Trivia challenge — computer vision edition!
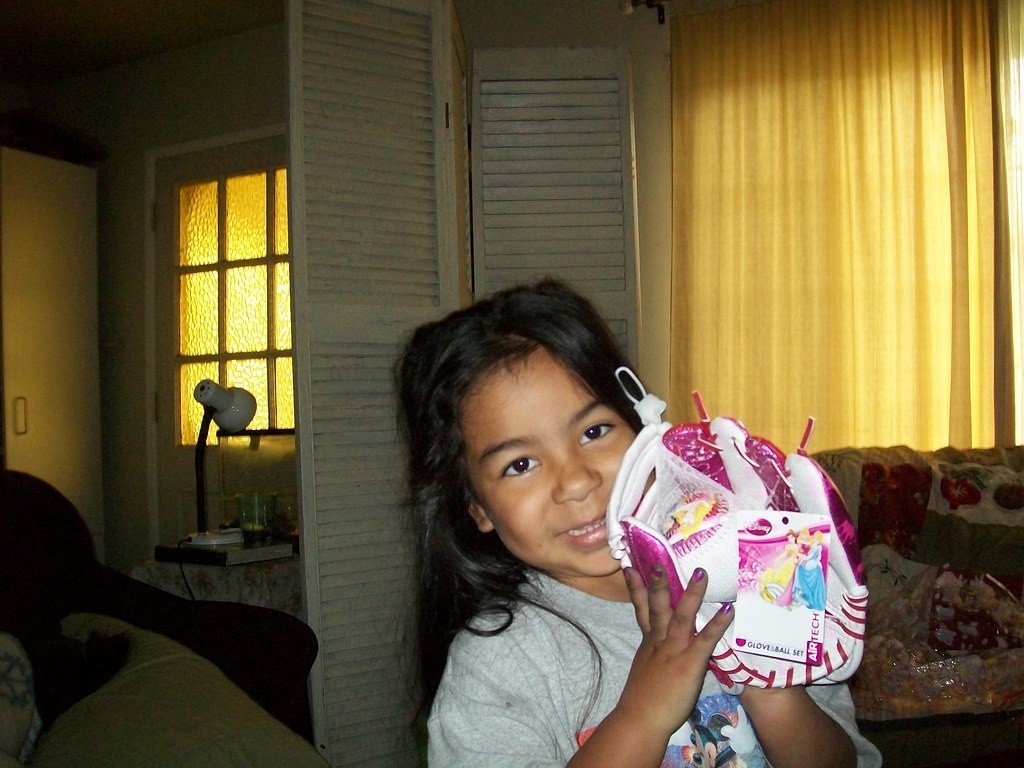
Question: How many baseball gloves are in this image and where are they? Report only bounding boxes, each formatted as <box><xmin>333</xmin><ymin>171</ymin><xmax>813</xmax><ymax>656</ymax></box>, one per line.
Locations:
<box><xmin>604</xmin><ymin>415</ymin><xmax>872</xmax><ymax>696</ymax></box>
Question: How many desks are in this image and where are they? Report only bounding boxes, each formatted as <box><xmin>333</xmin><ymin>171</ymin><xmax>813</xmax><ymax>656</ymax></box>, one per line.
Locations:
<box><xmin>143</xmin><ymin>556</ymin><xmax>302</xmax><ymax>617</ymax></box>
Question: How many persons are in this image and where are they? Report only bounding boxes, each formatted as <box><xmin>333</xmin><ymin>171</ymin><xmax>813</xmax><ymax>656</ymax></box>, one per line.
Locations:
<box><xmin>392</xmin><ymin>275</ymin><xmax>883</xmax><ymax>768</ymax></box>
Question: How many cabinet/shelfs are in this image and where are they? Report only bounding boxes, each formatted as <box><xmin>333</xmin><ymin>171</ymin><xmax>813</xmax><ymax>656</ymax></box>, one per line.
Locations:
<box><xmin>0</xmin><ymin>142</ymin><xmax>106</xmax><ymax>565</ymax></box>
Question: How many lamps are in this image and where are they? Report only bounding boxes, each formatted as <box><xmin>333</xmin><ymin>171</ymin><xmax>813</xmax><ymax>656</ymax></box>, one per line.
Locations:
<box><xmin>185</xmin><ymin>379</ymin><xmax>257</xmax><ymax>545</ymax></box>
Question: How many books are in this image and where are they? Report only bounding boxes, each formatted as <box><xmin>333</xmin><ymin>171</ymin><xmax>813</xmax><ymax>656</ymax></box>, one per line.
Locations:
<box><xmin>154</xmin><ymin>541</ymin><xmax>292</xmax><ymax>565</ymax></box>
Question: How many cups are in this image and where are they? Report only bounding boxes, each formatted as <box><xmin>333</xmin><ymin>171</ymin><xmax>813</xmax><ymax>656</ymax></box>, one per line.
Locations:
<box><xmin>274</xmin><ymin>488</ymin><xmax>297</xmax><ymax>533</ymax></box>
<box><xmin>234</xmin><ymin>492</ymin><xmax>272</xmax><ymax>546</ymax></box>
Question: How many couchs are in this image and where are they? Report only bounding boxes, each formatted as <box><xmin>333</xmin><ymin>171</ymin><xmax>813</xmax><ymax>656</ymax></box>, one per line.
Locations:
<box><xmin>808</xmin><ymin>444</ymin><xmax>1024</xmax><ymax>768</ymax></box>
<box><xmin>0</xmin><ymin>468</ymin><xmax>318</xmax><ymax>747</ymax></box>
